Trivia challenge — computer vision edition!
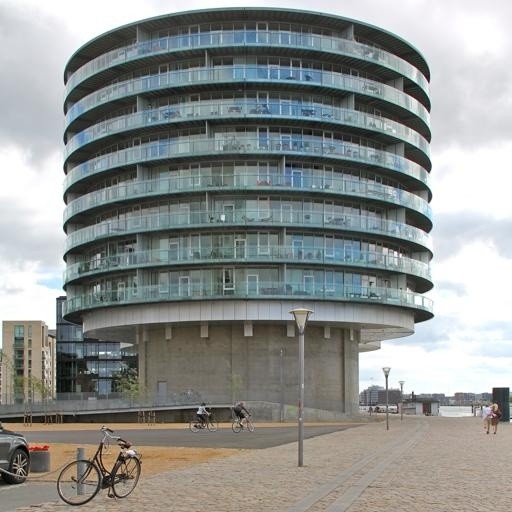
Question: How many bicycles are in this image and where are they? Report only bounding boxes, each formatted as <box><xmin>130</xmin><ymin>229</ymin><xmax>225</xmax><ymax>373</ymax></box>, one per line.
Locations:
<box><xmin>57</xmin><ymin>426</ymin><xmax>142</xmax><ymax>505</ymax></box>
<box><xmin>232</xmin><ymin>415</ymin><xmax>255</xmax><ymax>433</ymax></box>
<box><xmin>189</xmin><ymin>413</ymin><xmax>218</xmax><ymax>433</ymax></box>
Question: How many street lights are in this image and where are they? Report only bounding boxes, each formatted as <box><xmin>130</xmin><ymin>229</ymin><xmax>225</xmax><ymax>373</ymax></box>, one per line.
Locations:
<box><xmin>382</xmin><ymin>367</ymin><xmax>391</xmax><ymax>429</ymax></box>
<box><xmin>398</xmin><ymin>380</ymin><xmax>405</xmax><ymax>420</ymax></box>
<box><xmin>288</xmin><ymin>309</ymin><xmax>315</xmax><ymax>467</ymax></box>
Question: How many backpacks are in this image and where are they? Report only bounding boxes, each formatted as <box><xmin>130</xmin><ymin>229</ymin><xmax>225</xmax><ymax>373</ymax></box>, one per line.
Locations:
<box><xmin>233</xmin><ymin>407</ymin><xmax>245</xmax><ymax>417</ymax></box>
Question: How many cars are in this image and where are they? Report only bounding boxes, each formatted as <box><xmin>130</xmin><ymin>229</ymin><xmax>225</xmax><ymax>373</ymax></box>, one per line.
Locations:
<box><xmin>0</xmin><ymin>427</ymin><xmax>30</xmax><ymax>484</ymax></box>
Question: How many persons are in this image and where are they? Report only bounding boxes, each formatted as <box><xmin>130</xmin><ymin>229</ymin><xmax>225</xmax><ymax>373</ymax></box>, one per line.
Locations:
<box><xmin>481</xmin><ymin>404</ymin><xmax>496</xmax><ymax>434</ymax></box>
<box><xmin>233</xmin><ymin>400</ymin><xmax>251</xmax><ymax>428</ymax></box>
<box><xmin>491</xmin><ymin>403</ymin><xmax>502</xmax><ymax>434</ymax></box>
<box><xmin>196</xmin><ymin>402</ymin><xmax>211</xmax><ymax>429</ymax></box>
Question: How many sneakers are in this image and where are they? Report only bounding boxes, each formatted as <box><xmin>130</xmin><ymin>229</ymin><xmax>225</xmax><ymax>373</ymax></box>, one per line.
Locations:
<box><xmin>239</xmin><ymin>423</ymin><xmax>244</xmax><ymax>428</ymax></box>
